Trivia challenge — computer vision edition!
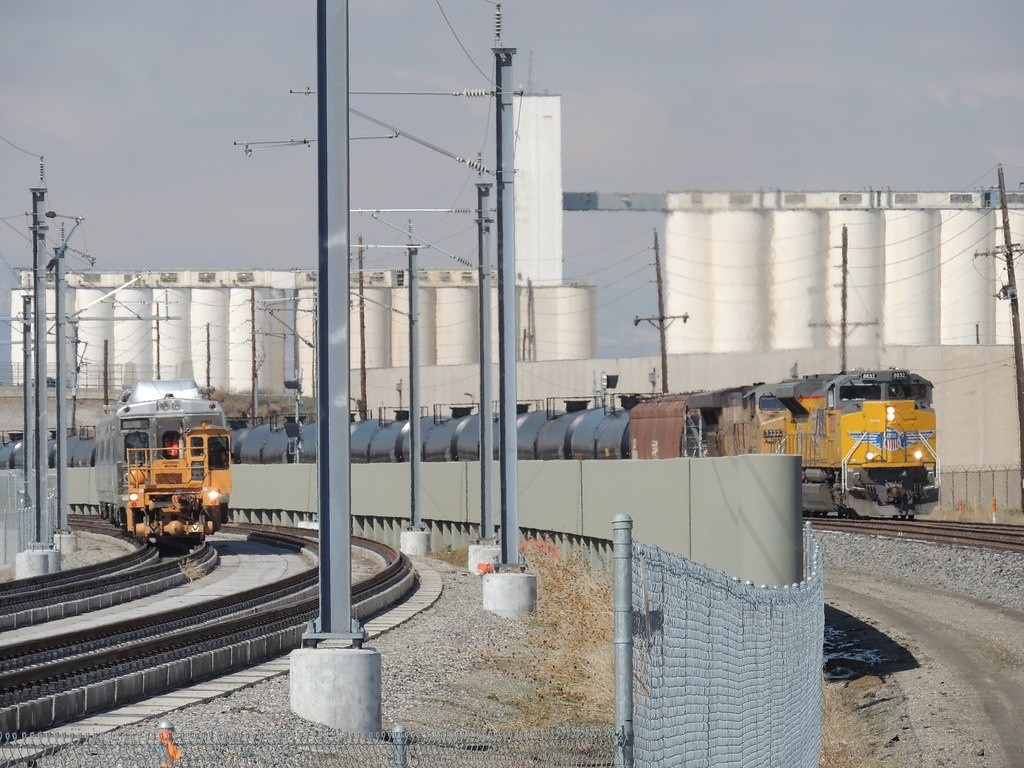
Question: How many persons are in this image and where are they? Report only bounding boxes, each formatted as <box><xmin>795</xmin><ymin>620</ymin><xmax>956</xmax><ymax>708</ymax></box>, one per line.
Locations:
<box><xmin>212</xmin><ymin>441</ymin><xmax>226</xmax><ymax>468</ymax></box>
<box><xmin>164</xmin><ymin>434</ymin><xmax>179</xmax><ymax>459</ymax></box>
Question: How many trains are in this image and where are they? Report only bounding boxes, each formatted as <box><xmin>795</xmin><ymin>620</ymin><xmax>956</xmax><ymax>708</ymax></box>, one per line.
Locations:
<box><xmin>0</xmin><ymin>364</ymin><xmax>941</xmax><ymax>519</ymax></box>
<box><xmin>95</xmin><ymin>378</ymin><xmax>234</xmax><ymax>545</ymax></box>
